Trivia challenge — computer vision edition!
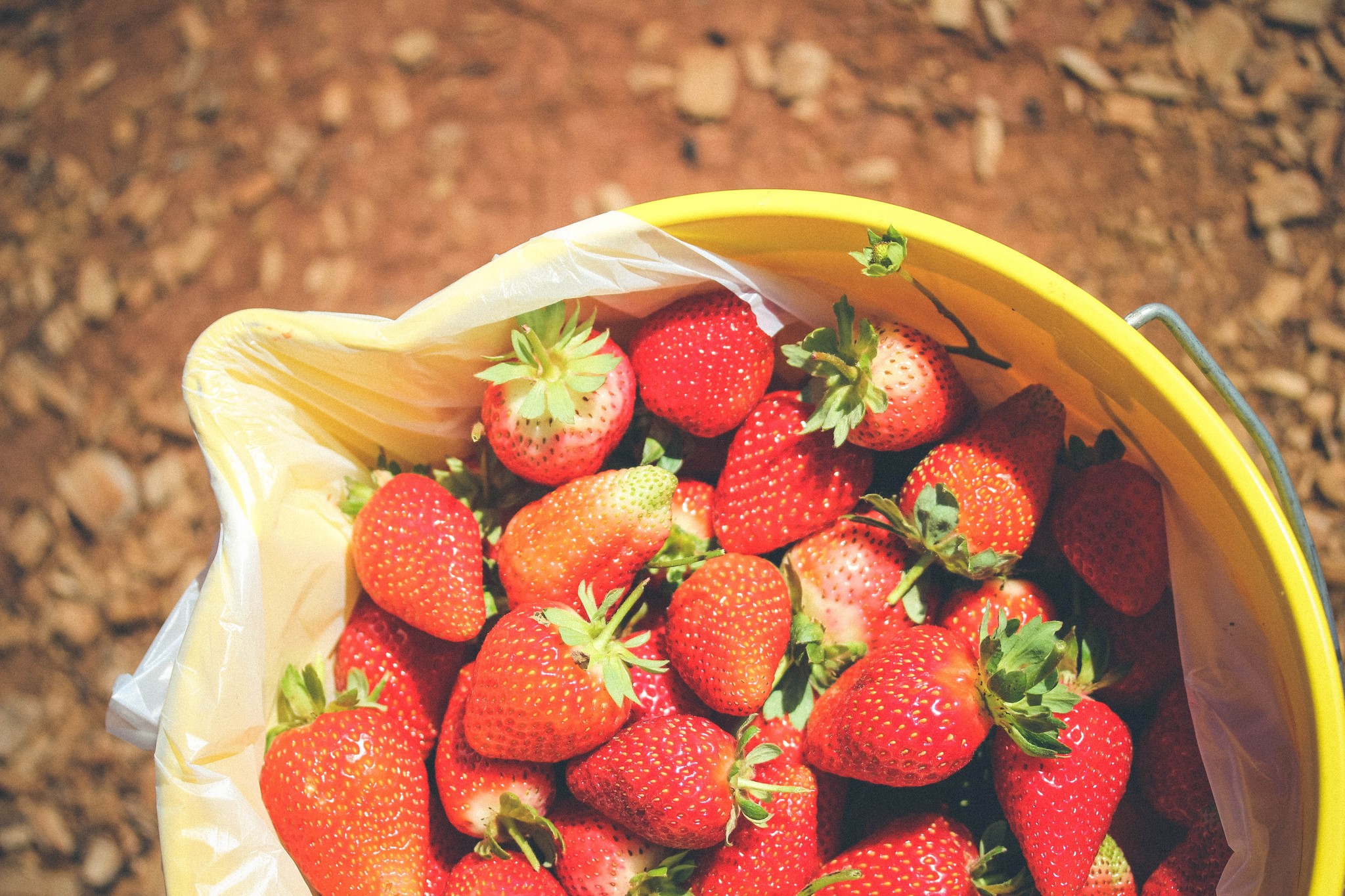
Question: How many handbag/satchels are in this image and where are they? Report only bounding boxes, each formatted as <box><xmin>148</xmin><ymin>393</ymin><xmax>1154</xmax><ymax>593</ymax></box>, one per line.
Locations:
<box><xmin>105</xmin><ymin>208</ymin><xmax>1305</xmax><ymax>894</ymax></box>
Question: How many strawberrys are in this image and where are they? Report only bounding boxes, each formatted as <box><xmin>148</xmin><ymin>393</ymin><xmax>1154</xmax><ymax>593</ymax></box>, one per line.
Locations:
<box><xmin>255</xmin><ymin>288</ymin><xmax>1239</xmax><ymax>896</ymax></box>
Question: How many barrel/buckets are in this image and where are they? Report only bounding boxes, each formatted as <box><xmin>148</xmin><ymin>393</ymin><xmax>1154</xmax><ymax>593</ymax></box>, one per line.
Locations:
<box><xmin>154</xmin><ymin>188</ymin><xmax>1345</xmax><ymax>896</ymax></box>
